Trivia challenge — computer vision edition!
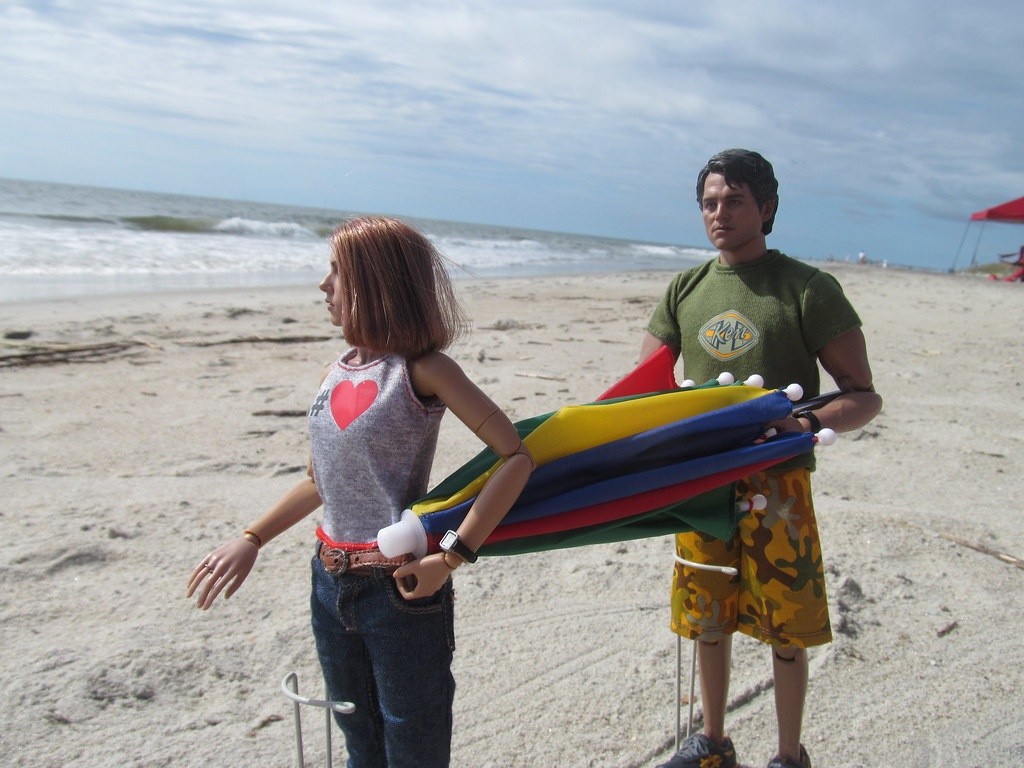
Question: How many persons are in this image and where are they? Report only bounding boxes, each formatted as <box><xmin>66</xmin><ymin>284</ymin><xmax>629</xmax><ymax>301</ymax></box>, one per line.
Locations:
<box><xmin>633</xmin><ymin>148</ymin><xmax>884</xmax><ymax>768</ymax></box>
<box><xmin>858</xmin><ymin>251</ymin><xmax>866</xmax><ymax>264</ymax></box>
<box><xmin>988</xmin><ymin>245</ymin><xmax>1024</xmax><ymax>282</ymax></box>
<box><xmin>188</xmin><ymin>217</ymin><xmax>537</xmax><ymax>767</ymax></box>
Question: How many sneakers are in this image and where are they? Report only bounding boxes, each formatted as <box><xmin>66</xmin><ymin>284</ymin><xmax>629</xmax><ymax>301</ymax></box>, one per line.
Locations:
<box><xmin>767</xmin><ymin>742</ymin><xmax>811</xmax><ymax>768</ymax></box>
<box><xmin>655</xmin><ymin>733</ymin><xmax>737</xmax><ymax>768</ymax></box>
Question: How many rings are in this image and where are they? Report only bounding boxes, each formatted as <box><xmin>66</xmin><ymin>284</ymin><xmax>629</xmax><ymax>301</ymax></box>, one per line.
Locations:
<box><xmin>204</xmin><ymin>564</ymin><xmax>213</xmax><ymax>573</ymax></box>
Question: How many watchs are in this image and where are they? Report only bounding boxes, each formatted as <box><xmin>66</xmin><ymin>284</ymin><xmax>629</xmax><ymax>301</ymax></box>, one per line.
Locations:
<box><xmin>440</xmin><ymin>529</ymin><xmax>478</xmax><ymax>564</ymax></box>
<box><xmin>792</xmin><ymin>411</ymin><xmax>822</xmax><ymax>434</ymax></box>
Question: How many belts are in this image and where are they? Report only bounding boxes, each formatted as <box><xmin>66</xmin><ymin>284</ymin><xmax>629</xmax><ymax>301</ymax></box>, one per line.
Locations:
<box><xmin>319</xmin><ymin>543</ymin><xmax>414</xmax><ymax>576</ymax></box>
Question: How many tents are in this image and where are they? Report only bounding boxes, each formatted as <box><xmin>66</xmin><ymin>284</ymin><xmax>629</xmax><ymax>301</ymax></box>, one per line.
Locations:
<box><xmin>950</xmin><ymin>197</ymin><xmax>1024</xmax><ymax>272</ymax></box>
